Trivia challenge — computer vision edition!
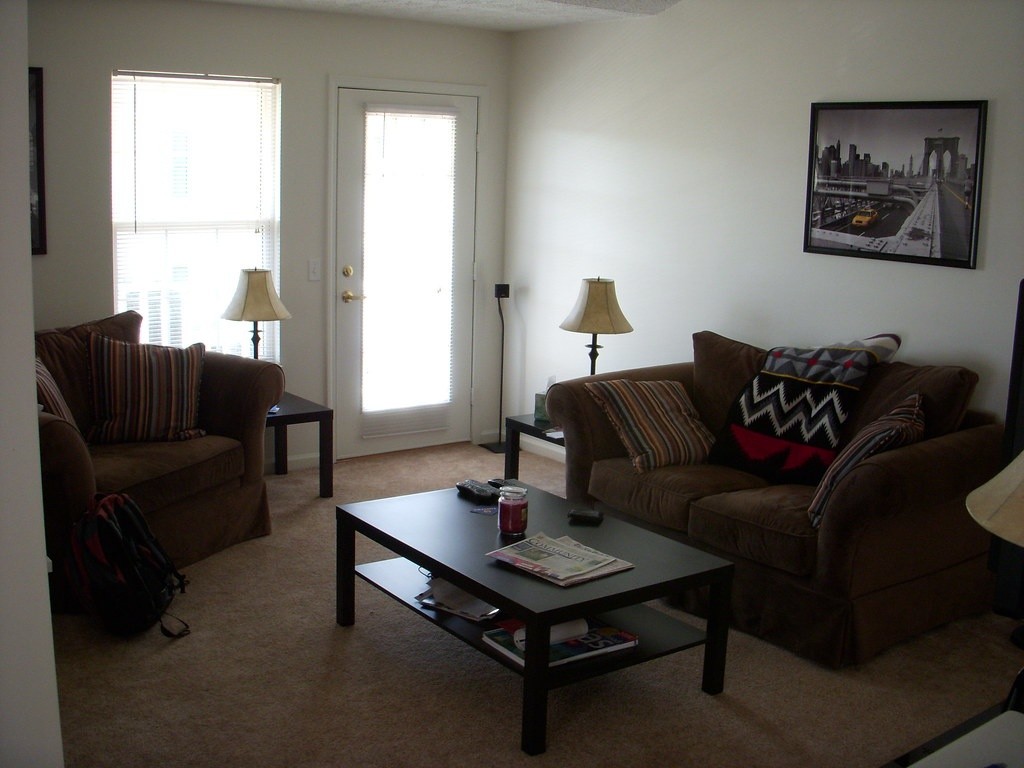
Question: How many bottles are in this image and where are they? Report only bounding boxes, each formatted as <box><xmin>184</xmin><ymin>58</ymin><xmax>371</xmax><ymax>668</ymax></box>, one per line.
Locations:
<box><xmin>498</xmin><ymin>485</ymin><xmax>528</xmax><ymax>535</ymax></box>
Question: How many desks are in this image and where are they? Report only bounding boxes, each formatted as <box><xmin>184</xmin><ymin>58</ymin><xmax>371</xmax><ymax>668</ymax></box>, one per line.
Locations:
<box><xmin>265</xmin><ymin>392</ymin><xmax>334</xmax><ymax>499</ymax></box>
<box><xmin>870</xmin><ymin>702</ymin><xmax>1024</xmax><ymax>768</ymax></box>
<box><xmin>503</xmin><ymin>415</ymin><xmax>567</xmax><ymax>478</ymax></box>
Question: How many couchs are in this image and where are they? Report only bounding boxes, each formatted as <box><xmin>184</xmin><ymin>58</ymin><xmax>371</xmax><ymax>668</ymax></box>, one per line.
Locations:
<box><xmin>545</xmin><ymin>331</ymin><xmax>1004</xmax><ymax>665</ymax></box>
<box><xmin>35</xmin><ymin>310</ymin><xmax>286</xmax><ymax>589</ymax></box>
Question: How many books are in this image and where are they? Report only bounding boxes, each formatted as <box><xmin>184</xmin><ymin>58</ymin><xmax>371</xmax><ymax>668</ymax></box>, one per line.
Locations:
<box><xmin>414</xmin><ymin>588</ymin><xmax>499</xmax><ymax>622</ymax></box>
<box><xmin>481</xmin><ymin>619</ymin><xmax>639</xmax><ymax>668</ymax></box>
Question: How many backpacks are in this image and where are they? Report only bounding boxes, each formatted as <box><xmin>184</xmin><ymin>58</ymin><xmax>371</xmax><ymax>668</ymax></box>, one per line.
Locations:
<box><xmin>72</xmin><ymin>493</ymin><xmax>191</xmax><ymax>639</ymax></box>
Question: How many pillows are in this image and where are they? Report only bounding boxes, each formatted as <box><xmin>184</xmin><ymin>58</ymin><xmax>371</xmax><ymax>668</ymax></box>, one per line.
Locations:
<box><xmin>35</xmin><ymin>354</ymin><xmax>77</xmax><ymax>429</ymax></box>
<box><xmin>583</xmin><ymin>378</ymin><xmax>716</xmax><ymax>475</ymax></box>
<box><xmin>84</xmin><ymin>331</ymin><xmax>208</xmax><ymax>444</ymax></box>
<box><xmin>807</xmin><ymin>393</ymin><xmax>922</xmax><ymax>529</ymax></box>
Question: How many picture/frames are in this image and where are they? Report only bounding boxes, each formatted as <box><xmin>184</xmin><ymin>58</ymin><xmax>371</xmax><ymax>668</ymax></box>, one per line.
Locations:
<box><xmin>802</xmin><ymin>100</ymin><xmax>988</xmax><ymax>268</ymax></box>
<box><xmin>28</xmin><ymin>66</ymin><xmax>47</xmax><ymax>258</ymax></box>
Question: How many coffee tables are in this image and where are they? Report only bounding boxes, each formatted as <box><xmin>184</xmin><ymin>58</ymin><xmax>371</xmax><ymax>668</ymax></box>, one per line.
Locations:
<box><xmin>335</xmin><ymin>477</ymin><xmax>734</xmax><ymax>757</ymax></box>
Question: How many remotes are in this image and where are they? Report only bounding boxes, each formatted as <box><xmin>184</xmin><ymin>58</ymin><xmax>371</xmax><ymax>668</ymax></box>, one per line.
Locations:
<box><xmin>455</xmin><ymin>478</ymin><xmax>520</xmax><ymax>502</ymax></box>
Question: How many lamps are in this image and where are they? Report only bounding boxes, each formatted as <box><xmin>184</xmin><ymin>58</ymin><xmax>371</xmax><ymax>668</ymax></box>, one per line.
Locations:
<box><xmin>965</xmin><ymin>448</ymin><xmax>1024</xmax><ymax>714</ymax></box>
<box><xmin>478</xmin><ymin>283</ymin><xmax>519</xmax><ymax>454</ymax></box>
<box><xmin>560</xmin><ymin>278</ymin><xmax>634</xmax><ymax>375</ymax></box>
<box><xmin>221</xmin><ymin>268</ymin><xmax>292</xmax><ymax>360</ymax></box>
